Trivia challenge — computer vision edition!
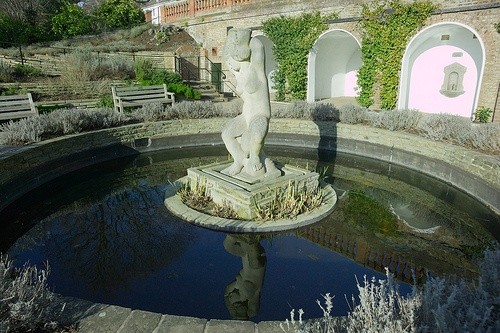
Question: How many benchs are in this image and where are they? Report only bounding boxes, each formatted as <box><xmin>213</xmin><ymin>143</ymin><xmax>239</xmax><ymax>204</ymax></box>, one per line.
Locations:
<box><xmin>0</xmin><ymin>93</ymin><xmax>39</xmax><ymax>121</ymax></box>
<box><xmin>111</xmin><ymin>83</ymin><xmax>176</xmax><ymax>116</ymax></box>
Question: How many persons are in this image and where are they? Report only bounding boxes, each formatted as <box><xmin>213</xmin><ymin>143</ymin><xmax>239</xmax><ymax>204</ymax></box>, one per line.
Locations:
<box><xmin>222</xmin><ymin>36</ymin><xmax>271</xmax><ymax>176</ymax></box>
